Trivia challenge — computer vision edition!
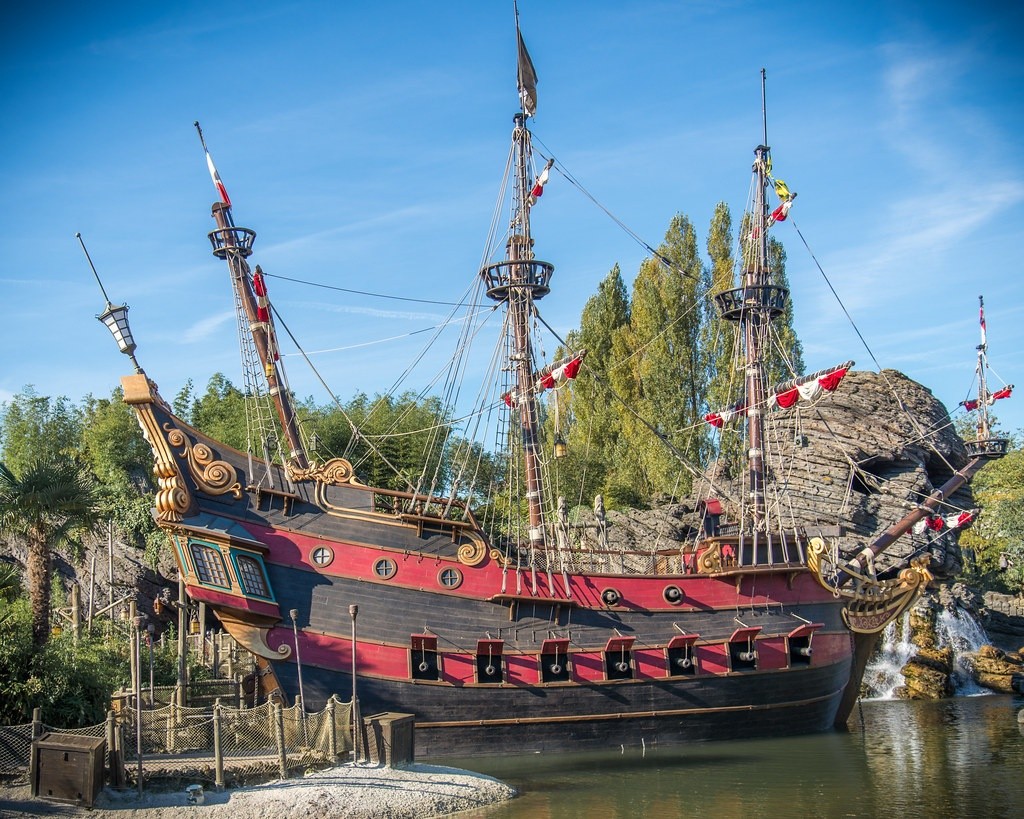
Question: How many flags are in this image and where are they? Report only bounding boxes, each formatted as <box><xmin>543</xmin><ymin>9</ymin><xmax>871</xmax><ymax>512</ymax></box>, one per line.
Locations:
<box><xmin>514</xmin><ymin>26</ymin><xmax>538</xmax><ymax>118</ymax></box>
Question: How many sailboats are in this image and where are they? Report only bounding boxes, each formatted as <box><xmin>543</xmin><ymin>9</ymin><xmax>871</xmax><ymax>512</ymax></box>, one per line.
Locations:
<box><xmin>75</xmin><ymin>1</ymin><xmax>1017</xmax><ymax>749</ymax></box>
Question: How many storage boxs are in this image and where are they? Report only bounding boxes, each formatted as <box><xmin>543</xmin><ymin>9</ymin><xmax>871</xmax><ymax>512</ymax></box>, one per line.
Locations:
<box><xmin>31</xmin><ymin>732</ymin><xmax>106</xmax><ymax>808</ymax></box>
<box><xmin>361</xmin><ymin>712</ymin><xmax>415</xmax><ymax>768</ymax></box>
